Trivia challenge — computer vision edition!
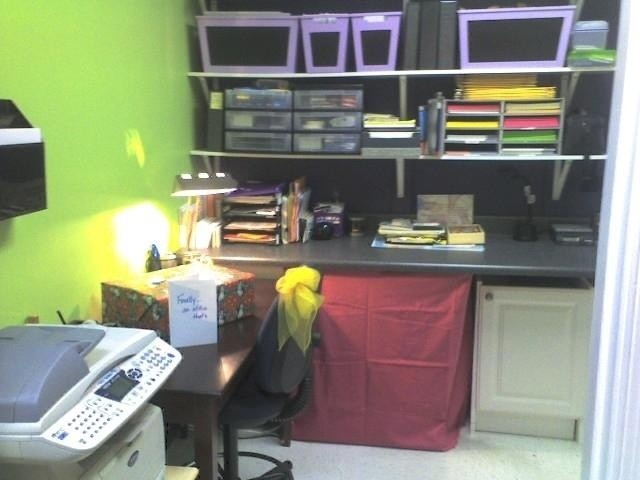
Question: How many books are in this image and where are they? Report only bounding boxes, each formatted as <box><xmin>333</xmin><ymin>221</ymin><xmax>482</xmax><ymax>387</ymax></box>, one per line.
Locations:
<box><xmin>363</xmin><ymin>113</ymin><xmax>417</xmax><ymax>139</ymax></box>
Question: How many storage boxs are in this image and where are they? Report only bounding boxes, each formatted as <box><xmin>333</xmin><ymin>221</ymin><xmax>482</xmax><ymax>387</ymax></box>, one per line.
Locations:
<box><xmin>102</xmin><ymin>261</ymin><xmax>255</xmax><ymax>340</ymax></box>
<box><xmin>350</xmin><ymin>12</ymin><xmax>404</xmax><ymax>71</ymax></box>
<box><xmin>196</xmin><ymin>11</ymin><xmax>299</xmax><ymax>72</ymax></box>
<box><xmin>457</xmin><ymin>6</ymin><xmax>578</xmax><ymax>69</ymax></box>
<box><xmin>298</xmin><ymin>13</ymin><xmax>350</xmax><ymax>74</ymax></box>
<box><xmin>566</xmin><ymin>21</ymin><xmax>609</xmax><ymax>65</ymax></box>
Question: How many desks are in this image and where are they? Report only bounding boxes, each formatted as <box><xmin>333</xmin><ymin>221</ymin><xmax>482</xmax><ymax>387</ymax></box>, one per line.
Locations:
<box><xmin>92</xmin><ymin>279</ymin><xmax>280</xmax><ymax>479</ymax></box>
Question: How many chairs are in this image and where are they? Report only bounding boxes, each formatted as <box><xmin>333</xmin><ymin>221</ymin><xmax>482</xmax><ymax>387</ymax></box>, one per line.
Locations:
<box><xmin>217</xmin><ymin>266</ymin><xmax>325</xmax><ymax>480</ymax></box>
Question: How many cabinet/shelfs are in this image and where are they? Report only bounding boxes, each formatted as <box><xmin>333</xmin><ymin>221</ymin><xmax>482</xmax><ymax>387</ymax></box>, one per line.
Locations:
<box><xmin>472</xmin><ymin>275</ymin><xmax>598</xmax><ymax>443</ymax></box>
<box><xmin>187</xmin><ymin>66</ymin><xmax>614</xmax><ymax>220</ymax></box>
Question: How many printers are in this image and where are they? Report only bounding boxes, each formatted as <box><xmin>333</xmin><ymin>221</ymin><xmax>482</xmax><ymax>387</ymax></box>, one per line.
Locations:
<box><xmin>0</xmin><ymin>323</ymin><xmax>183</xmax><ymax>480</ymax></box>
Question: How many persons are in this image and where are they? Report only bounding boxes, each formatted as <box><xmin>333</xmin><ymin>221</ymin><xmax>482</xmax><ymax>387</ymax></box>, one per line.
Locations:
<box><xmin>222</xmin><ymin>176</ymin><xmax>315</xmax><ymax>245</ymax></box>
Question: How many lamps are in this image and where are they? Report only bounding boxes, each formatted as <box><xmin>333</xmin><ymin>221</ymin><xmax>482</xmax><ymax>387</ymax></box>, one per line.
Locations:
<box><xmin>172</xmin><ymin>172</ymin><xmax>239</xmax><ymax>257</ymax></box>
